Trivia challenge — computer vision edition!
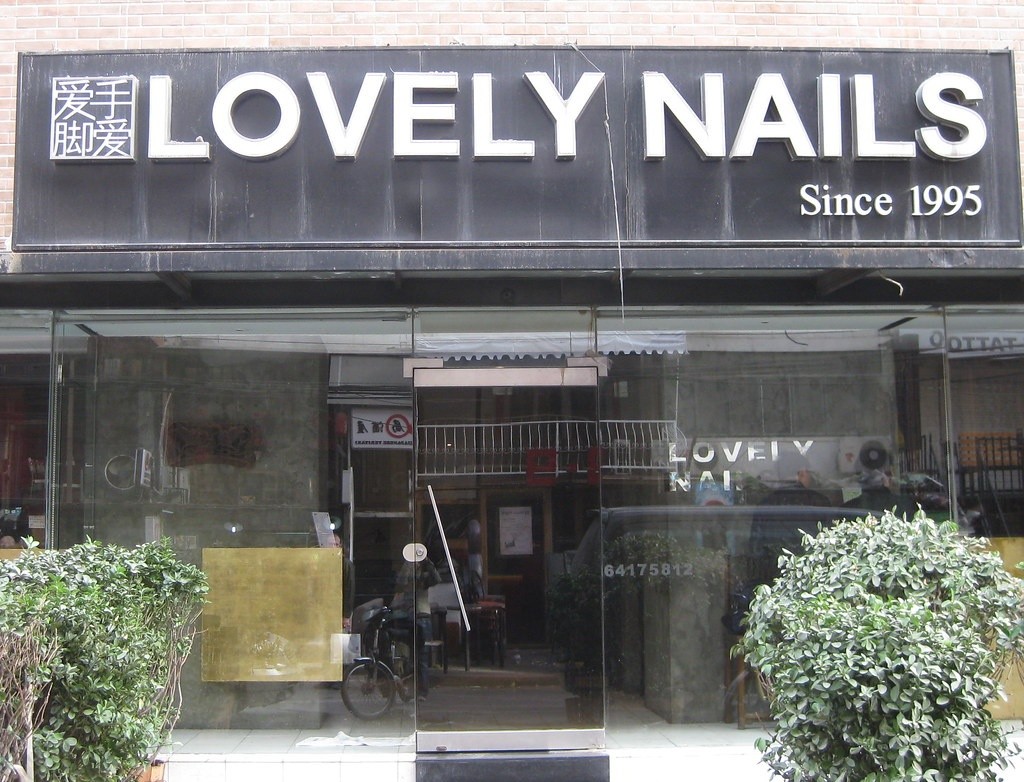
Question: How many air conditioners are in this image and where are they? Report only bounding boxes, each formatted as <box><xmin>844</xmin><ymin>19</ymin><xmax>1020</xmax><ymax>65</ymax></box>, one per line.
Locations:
<box><xmin>838</xmin><ymin>435</ymin><xmax>893</xmax><ymax>472</ymax></box>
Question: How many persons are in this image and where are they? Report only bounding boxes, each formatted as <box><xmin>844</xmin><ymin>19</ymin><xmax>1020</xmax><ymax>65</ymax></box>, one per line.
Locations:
<box><xmin>736</xmin><ymin>451</ymin><xmax>973</xmax><ymax>712</ymax></box>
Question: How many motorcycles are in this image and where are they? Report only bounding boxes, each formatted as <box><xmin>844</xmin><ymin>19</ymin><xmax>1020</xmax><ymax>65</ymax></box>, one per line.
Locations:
<box><xmin>340</xmin><ymin>605</ymin><xmax>415</xmax><ymax>721</ymax></box>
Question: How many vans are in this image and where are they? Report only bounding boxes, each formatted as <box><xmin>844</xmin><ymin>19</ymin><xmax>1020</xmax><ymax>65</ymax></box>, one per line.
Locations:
<box><xmin>573</xmin><ymin>503</ymin><xmax>885</xmax><ymax>577</ymax></box>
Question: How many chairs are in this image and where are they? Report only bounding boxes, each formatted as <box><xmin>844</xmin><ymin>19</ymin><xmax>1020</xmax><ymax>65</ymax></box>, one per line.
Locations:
<box><xmin>461</xmin><ymin>571</ymin><xmax>507</xmax><ymax>668</ymax></box>
<box><xmin>725</xmin><ymin>548</ymin><xmax>780</xmax><ymax>730</ymax></box>
